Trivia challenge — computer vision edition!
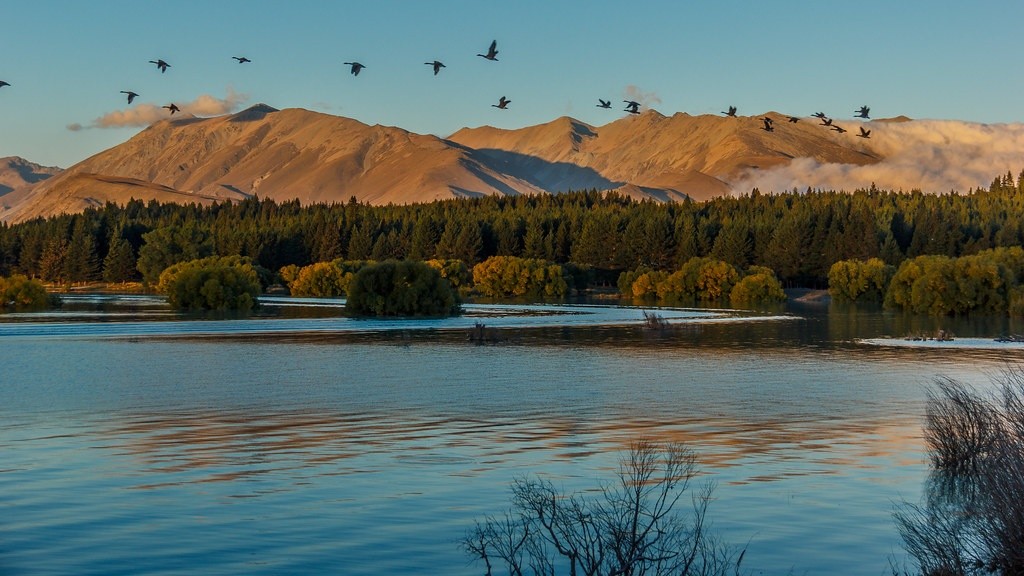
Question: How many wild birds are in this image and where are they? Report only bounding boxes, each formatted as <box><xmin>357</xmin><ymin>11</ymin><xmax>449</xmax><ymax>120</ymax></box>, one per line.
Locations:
<box><xmin>342</xmin><ymin>39</ymin><xmax>513</xmax><ymax>111</ymax></box>
<box><xmin>993</xmin><ymin>334</ymin><xmax>1024</xmax><ymax>343</ymax></box>
<box><xmin>1</xmin><ymin>55</ymin><xmax>252</xmax><ymax>116</ymax></box>
<box><xmin>902</xmin><ymin>328</ymin><xmax>956</xmax><ymax>342</ymax></box>
<box><xmin>595</xmin><ymin>98</ymin><xmax>642</xmax><ymax>115</ymax></box>
<box><xmin>719</xmin><ymin>104</ymin><xmax>871</xmax><ymax>139</ymax></box>
